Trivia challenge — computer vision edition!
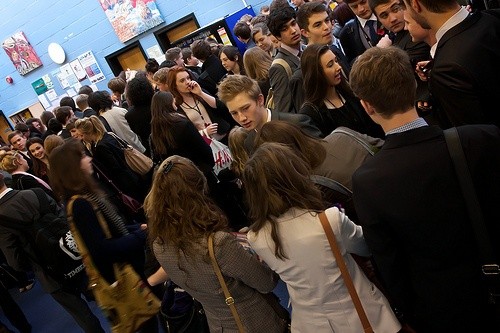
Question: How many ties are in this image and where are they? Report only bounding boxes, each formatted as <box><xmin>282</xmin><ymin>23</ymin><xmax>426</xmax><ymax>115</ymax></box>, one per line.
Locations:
<box><xmin>366</xmin><ymin>21</ymin><xmax>379</xmax><ymax>46</ymax></box>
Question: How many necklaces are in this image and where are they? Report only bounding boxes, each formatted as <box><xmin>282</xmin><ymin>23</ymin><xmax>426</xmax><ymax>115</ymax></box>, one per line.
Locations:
<box><xmin>326</xmin><ymin>92</ymin><xmax>352</xmax><ymax>123</ymax></box>
<box><xmin>184</xmin><ymin>97</ymin><xmax>209</xmax><ymax>127</ymax></box>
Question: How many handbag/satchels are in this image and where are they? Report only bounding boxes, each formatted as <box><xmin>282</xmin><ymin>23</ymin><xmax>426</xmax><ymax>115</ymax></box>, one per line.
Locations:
<box><xmin>121</xmin><ymin>145</ymin><xmax>154</xmax><ymax>176</ymax></box>
<box><xmin>203</xmin><ymin>129</ymin><xmax>233</xmax><ymax>176</ymax></box>
<box><xmin>120</xmin><ymin>194</ymin><xmax>143</xmax><ymax>217</ymax></box>
<box><xmin>89</xmin><ymin>265</ymin><xmax>163</xmax><ymax>333</ymax></box>
<box><xmin>264</xmin><ymin>88</ymin><xmax>276</xmax><ymax>110</ymax></box>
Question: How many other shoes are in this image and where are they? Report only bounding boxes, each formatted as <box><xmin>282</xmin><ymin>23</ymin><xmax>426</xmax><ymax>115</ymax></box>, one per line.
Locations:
<box><xmin>18</xmin><ymin>282</ymin><xmax>34</xmax><ymax>292</ymax></box>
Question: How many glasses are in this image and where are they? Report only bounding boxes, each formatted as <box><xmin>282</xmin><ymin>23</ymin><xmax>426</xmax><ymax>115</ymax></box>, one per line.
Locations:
<box><xmin>247</xmin><ymin>23</ymin><xmax>250</xmax><ymax>26</ymax></box>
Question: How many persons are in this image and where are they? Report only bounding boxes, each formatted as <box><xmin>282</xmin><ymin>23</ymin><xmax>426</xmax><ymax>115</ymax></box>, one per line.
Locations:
<box><xmin>0</xmin><ymin>0</ymin><xmax>500</xmax><ymax>333</ymax></box>
